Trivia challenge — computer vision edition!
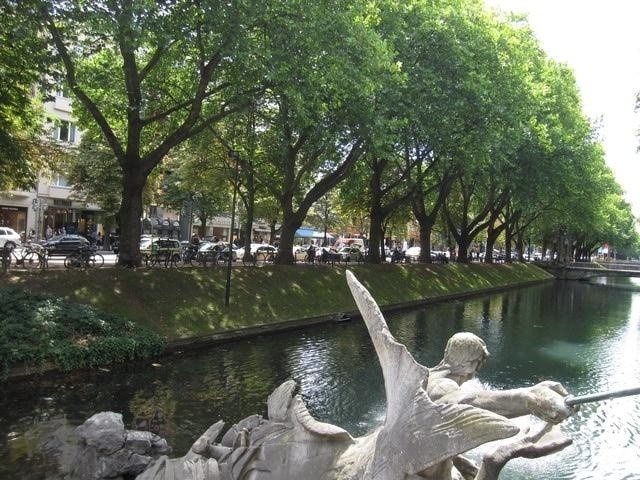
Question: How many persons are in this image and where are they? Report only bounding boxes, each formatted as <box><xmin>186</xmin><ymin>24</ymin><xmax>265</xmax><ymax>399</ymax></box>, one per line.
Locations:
<box><xmin>390</xmin><ymin>246</ymin><xmax>405</xmax><ymax>264</ymax></box>
<box><xmin>0</xmin><ymin>220</ymin><xmax>6</xmax><ymax>226</ymax></box>
<box><xmin>19</xmin><ymin>224</ymin><xmax>103</xmax><ymax>250</ymax></box>
<box><xmin>292</xmin><ymin>238</ymin><xmax>364</xmax><ymax>262</ymax></box>
<box><xmin>253</xmin><ymin>233</ymin><xmax>264</xmax><ymax>244</ymax></box>
<box><xmin>5</xmin><ymin>220</ymin><xmax>12</xmax><ymax>228</ymax></box>
<box><xmin>191</xmin><ymin>232</ymin><xmax>241</xmax><ymax>253</ymax></box>
<box><xmin>418</xmin><ymin>332</ymin><xmax>579</xmax><ymax>480</ymax></box>
<box><xmin>431</xmin><ymin>248</ymin><xmax>550</xmax><ymax>265</ymax></box>
